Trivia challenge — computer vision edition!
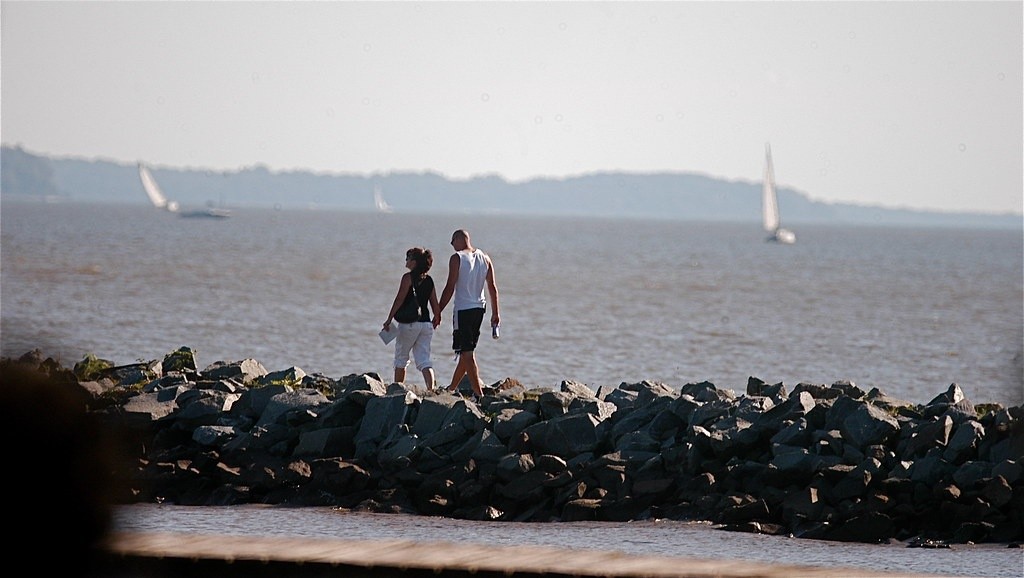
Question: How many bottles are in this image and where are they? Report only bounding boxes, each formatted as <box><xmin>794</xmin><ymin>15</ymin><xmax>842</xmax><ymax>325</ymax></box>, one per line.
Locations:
<box><xmin>493</xmin><ymin>323</ymin><xmax>499</xmax><ymax>339</ymax></box>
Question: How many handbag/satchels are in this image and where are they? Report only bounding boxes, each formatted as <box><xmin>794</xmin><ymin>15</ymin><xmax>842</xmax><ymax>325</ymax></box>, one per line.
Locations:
<box><xmin>394</xmin><ymin>273</ymin><xmax>421</xmax><ymax>324</ymax></box>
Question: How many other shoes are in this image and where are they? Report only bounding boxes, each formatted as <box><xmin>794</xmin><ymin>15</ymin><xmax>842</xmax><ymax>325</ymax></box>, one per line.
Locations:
<box><xmin>470</xmin><ymin>394</ymin><xmax>485</xmax><ymax>406</ymax></box>
<box><xmin>444</xmin><ymin>385</ymin><xmax>455</xmax><ymax>394</ymax></box>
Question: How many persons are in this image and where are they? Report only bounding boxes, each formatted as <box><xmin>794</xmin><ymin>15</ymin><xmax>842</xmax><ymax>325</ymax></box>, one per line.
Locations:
<box><xmin>431</xmin><ymin>229</ymin><xmax>501</xmax><ymax>399</ymax></box>
<box><xmin>383</xmin><ymin>247</ymin><xmax>441</xmax><ymax>390</ymax></box>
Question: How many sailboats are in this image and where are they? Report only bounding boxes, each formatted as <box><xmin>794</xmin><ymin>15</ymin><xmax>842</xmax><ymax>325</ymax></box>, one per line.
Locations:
<box><xmin>760</xmin><ymin>145</ymin><xmax>796</xmax><ymax>244</ymax></box>
<box><xmin>136</xmin><ymin>163</ymin><xmax>178</xmax><ymax>213</ymax></box>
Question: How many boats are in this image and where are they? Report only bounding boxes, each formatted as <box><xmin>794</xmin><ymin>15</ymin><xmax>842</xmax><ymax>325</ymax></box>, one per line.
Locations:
<box><xmin>181</xmin><ymin>210</ymin><xmax>229</xmax><ymax>219</ymax></box>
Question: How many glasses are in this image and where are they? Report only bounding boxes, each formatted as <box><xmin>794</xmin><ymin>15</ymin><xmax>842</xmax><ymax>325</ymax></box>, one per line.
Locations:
<box><xmin>451</xmin><ymin>237</ymin><xmax>456</xmax><ymax>246</ymax></box>
<box><xmin>405</xmin><ymin>257</ymin><xmax>410</xmax><ymax>261</ymax></box>
